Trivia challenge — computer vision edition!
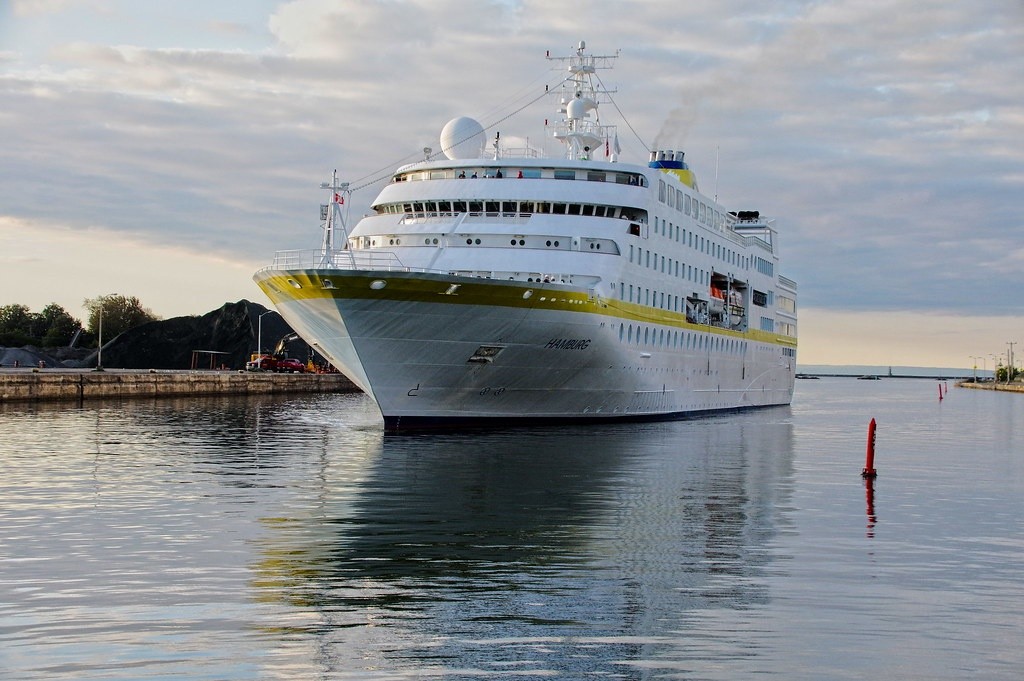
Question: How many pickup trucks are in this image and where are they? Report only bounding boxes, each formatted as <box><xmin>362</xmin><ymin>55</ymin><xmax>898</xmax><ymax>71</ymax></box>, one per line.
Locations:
<box><xmin>277</xmin><ymin>359</ymin><xmax>304</xmax><ymax>373</ymax></box>
<box><xmin>246</xmin><ymin>358</ymin><xmax>277</xmax><ymax>373</ymax></box>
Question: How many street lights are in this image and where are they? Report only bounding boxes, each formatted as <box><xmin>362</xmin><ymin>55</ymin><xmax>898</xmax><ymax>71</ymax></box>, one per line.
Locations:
<box><xmin>258</xmin><ymin>311</ymin><xmax>272</xmax><ymax>368</ymax></box>
<box><xmin>969</xmin><ymin>352</ymin><xmax>1024</xmax><ymax>384</ymax></box>
<box><xmin>96</xmin><ymin>293</ymin><xmax>117</xmax><ymax>371</ymax></box>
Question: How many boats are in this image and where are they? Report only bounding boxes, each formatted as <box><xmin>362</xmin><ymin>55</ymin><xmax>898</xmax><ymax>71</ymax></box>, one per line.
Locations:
<box><xmin>252</xmin><ymin>37</ymin><xmax>798</xmax><ymax>434</ymax></box>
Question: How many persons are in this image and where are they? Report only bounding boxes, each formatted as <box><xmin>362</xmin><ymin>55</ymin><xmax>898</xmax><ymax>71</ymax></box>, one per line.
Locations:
<box><xmin>496</xmin><ymin>167</ymin><xmax>502</xmax><ymax>178</ymax></box>
<box><xmin>471</xmin><ymin>172</ymin><xmax>494</xmax><ymax>178</ymax></box>
<box><xmin>517</xmin><ymin>171</ymin><xmax>524</xmax><ymax>178</ymax></box>
<box><xmin>459</xmin><ymin>171</ymin><xmax>466</xmax><ymax>179</ymax></box>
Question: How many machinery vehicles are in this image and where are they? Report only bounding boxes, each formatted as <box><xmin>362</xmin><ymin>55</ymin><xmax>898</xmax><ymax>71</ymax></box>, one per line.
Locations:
<box><xmin>251</xmin><ymin>331</ymin><xmax>316</xmax><ymax>373</ymax></box>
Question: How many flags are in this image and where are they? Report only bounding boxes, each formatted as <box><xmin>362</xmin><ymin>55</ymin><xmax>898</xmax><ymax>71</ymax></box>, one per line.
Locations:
<box><xmin>606</xmin><ymin>135</ymin><xmax>610</xmax><ymax>157</ymax></box>
<box><xmin>613</xmin><ymin>133</ymin><xmax>621</xmax><ymax>156</ymax></box>
<box><xmin>334</xmin><ymin>192</ymin><xmax>345</xmax><ymax>204</ymax></box>
